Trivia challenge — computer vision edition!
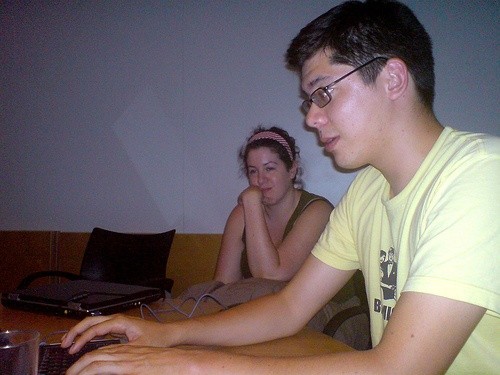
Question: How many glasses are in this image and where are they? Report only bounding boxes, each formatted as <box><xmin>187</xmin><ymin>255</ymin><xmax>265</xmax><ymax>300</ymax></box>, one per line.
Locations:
<box><xmin>302</xmin><ymin>56</ymin><xmax>391</xmax><ymax>114</ymax></box>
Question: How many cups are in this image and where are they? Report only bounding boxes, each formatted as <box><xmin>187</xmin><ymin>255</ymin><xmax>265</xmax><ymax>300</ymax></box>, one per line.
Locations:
<box><xmin>0</xmin><ymin>330</ymin><xmax>39</xmax><ymax>375</ymax></box>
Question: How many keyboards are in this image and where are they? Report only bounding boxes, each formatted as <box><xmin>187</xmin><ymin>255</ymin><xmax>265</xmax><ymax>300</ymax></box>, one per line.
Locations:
<box><xmin>37</xmin><ymin>339</ymin><xmax>124</xmax><ymax>375</ymax></box>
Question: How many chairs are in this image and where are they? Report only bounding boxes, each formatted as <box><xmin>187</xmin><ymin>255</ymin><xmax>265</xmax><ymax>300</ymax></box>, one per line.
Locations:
<box><xmin>17</xmin><ymin>228</ymin><xmax>176</xmax><ymax>291</ymax></box>
<box><xmin>323</xmin><ymin>305</ymin><xmax>373</xmax><ymax>351</ymax></box>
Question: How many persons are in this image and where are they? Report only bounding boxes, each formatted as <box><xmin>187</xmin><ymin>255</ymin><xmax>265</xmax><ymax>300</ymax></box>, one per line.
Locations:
<box><xmin>176</xmin><ymin>126</ymin><xmax>336</xmax><ymax>306</ymax></box>
<box><xmin>60</xmin><ymin>0</ymin><xmax>500</xmax><ymax>375</ymax></box>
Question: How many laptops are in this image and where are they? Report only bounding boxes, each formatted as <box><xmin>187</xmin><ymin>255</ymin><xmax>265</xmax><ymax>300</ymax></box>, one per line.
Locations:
<box><xmin>2</xmin><ymin>280</ymin><xmax>163</xmax><ymax>321</ymax></box>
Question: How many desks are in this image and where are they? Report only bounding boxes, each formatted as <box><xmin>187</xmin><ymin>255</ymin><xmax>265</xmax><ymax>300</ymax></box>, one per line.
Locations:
<box><xmin>0</xmin><ymin>298</ymin><xmax>359</xmax><ymax>375</ymax></box>
